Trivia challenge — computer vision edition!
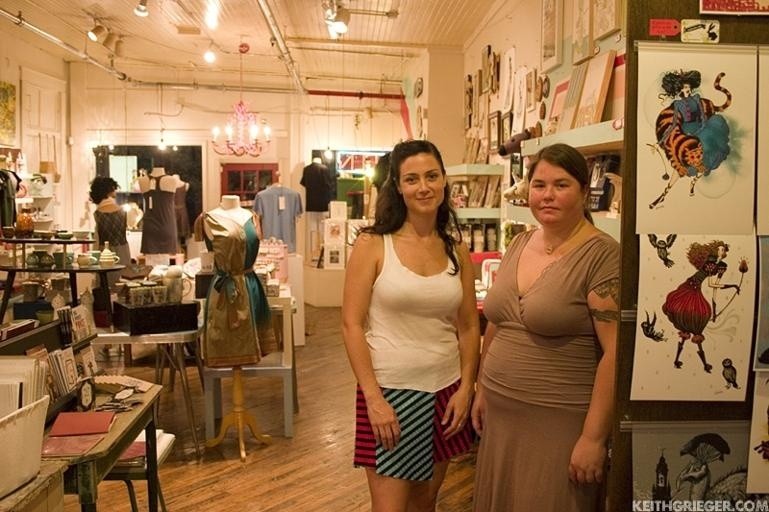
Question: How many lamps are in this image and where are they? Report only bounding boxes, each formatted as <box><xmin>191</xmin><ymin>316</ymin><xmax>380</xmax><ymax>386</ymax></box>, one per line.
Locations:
<box><xmin>211</xmin><ymin>44</ymin><xmax>274</xmax><ymax>158</ymax></box>
<box><xmin>154</xmin><ymin>86</ymin><xmax>168</xmax><ymax>152</ymax></box>
<box><xmin>85</xmin><ymin>18</ymin><xmax>133</xmax><ymax>60</ymax></box>
<box><xmin>129</xmin><ymin>0</ymin><xmax>153</xmax><ymax>20</ymax></box>
<box><xmin>324</xmin><ymin>44</ymin><xmax>333</xmax><ymax>162</ymax></box>
<box><xmin>321</xmin><ymin>0</ymin><xmax>400</xmax><ymax>43</ymax></box>
<box><xmin>364</xmin><ymin>81</ymin><xmax>376</xmax><ymax>177</ymax></box>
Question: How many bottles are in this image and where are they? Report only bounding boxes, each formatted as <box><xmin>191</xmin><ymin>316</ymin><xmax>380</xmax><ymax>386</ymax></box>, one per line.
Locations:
<box><xmin>14</xmin><ymin>206</ymin><xmax>35</xmax><ymax>238</ymax></box>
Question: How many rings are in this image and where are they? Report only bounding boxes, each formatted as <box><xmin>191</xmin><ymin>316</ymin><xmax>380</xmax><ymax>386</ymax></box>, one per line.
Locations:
<box><xmin>458</xmin><ymin>423</ymin><xmax>464</xmax><ymax>427</ymax></box>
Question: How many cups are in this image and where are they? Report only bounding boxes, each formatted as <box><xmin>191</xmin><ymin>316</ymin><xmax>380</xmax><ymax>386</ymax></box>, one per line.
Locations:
<box><xmin>67</xmin><ymin>252</ymin><xmax>74</xmax><ymax>263</ymax></box>
<box><xmin>77</xmin><ymin>254</ymin><xmax>97</xmax><ymax>269</ymax></box>
<box><xmin>87</xmin><ymin>250</ymin><xmax>101</xmax><ymax>265</ymax></box>
<box><xmin>162</xmin><ymin>275</ymin><xmax>192</xmax><ymax>304</ymax></box>
<box><xmin>53</xmin><ymin>252</ymin><xmax>70</xmax><ymax>268</ymax></box>
<box><xmin>99</xmin><ymin>249</ymin><xmax>120</xmax><ymax>269</ymax></box>
<box><xmin>21</xmin><ymin>281</ymin><xmax>45</xmax><ymax>301</ymax></box>
<box><xmin>3</xmin><ymin>227</ymin><xmax>14</xmax><ymax>237</ymax></box>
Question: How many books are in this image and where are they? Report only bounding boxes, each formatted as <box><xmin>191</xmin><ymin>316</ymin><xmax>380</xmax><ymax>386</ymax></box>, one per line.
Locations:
<box><xmin>0</xmin><ymin>303</ymin><xmax>165</xmax><ymax>470</ymax></box>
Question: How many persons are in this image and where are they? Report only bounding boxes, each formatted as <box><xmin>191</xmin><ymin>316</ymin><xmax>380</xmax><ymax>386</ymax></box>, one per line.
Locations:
<box><xmin>299</xmin><ymin>156</ymin><xmax>334</xmax><ymax>262</ymax></box>
<box><xmin>336</xmin><ymin>138</ymin><xmax>483</xmax><ymax>512</ymax></box>
<box><xmin>139</xmin><ymin>166</ymin><xmax>179</xmax><ymax>257</ymax></box>
<box><xmin>168</xmin><ymin>173</ymin><xmax>194</xmax><ymax>240</ymax></box>
<box><xmin>88</xmin><ymin>176</ymin><xmax>132</xmax><ymax>271</ymax></box>
<box><xmin>648</xmin><ymin>70</ymin><xmax>731</xmax><ymax>210</ymax></box>
<box><xmin>470</xmin><ymin>142</ymin><xmax>625</xmax><ymax>512</ymax></box>
<box><xmin>662</xmin><ymin>240</ymin><xmax>741</xmax><ymax>373</ymax></box>
<box><xmin>193</xmin><ymin>195</ymin><xmax>279</xmax><ymax>369</ymax></box>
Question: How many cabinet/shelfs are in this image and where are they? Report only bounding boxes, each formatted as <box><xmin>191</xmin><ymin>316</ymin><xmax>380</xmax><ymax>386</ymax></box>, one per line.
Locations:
<box><xmin>0</xmin><ymin>190</ymin><xmax>303</xmax><ymax>510</ymax></box>
<box><xmin>441</xmin><ymin>163</ymin><xmax>502</xmax><ymax>257</ymax></box>
<box><xmin>500</xmin><ymin>120</ymin><xmax>633</xmax><ymax>264</ymax></box>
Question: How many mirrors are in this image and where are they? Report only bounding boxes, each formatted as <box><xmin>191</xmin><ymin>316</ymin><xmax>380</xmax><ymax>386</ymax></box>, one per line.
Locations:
<box><xmin>92</xmin><ymin>142</ymin><xmax>203</xmax><ymax>234</ymax></box>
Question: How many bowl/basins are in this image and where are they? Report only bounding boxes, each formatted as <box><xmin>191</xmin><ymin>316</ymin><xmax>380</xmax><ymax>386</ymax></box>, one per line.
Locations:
<box><xmin>33</xmin><ymin>230</ymin><xmax>90</xmax><ymax>240</ymax></box>
<box><xmin>36</xmin><ymin>310</ymin><xmax>53</xmax><ymax>324</ymax></box>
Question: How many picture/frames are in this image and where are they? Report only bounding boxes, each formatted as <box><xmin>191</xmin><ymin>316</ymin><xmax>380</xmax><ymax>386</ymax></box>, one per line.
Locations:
<box><xmin>462</xmin><ymin>42</ymin><xmax>551</xmax><ymax>160</ymax></box>
